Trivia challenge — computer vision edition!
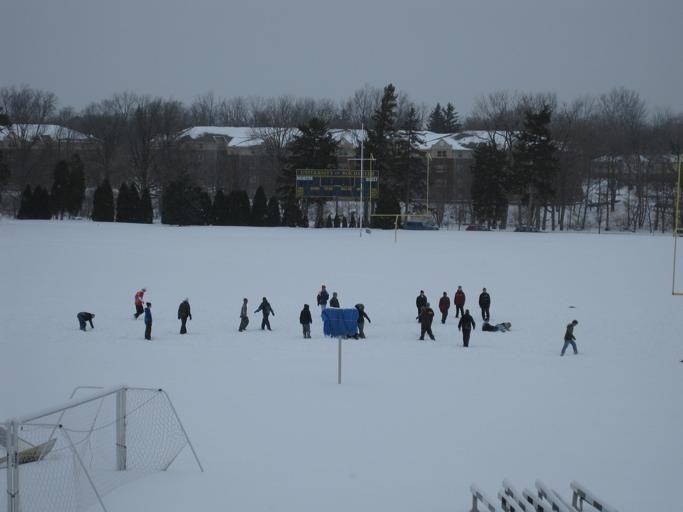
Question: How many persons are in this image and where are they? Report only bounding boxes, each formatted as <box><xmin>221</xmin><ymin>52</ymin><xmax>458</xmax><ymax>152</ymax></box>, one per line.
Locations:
<box><xmin>439</xmin><ymin>291</ymin><xmax>450</xmax><ymax>323</ymax></box>
<box><xmin>77</xmin><ymin>312</ymin><xmax>95</xmax><ymax>330</ymax></box>
<box><xmin>133</xmin><ymin>288</ymin><xmax>146</xmax><ymax>319</ymax></box>
<box><xmin>144</xmin><ymin>302</ymin><xmax>153</xmax><ymax>339</ymax></box>
<box><xmin>300</xmin><ymin>304</ymin><xmax>312</xmax><ymax>338</ymax></box>
<box><xmin>482</xmin><ymin>322</ymin><xmax>511</xmax><ymax>332</ymax></box>
<box><xmin>238</xmin><ymin>298</ymin><xmax>249</xmax><ymax>331</ymax></box>
<box><xmin>454</xmin><ymin>286</ymin><xmax>465</xmax><ymax>317</ymax></box>
<box><xmin>178</xmin><ymin>297</ymin><xmax>192</xmax><ymax>334</ymax></box>
<box><xmin>254</xmin><ymin>297</ymin><xmax>274</xmax><ymax>330</ymax></box>
<box><xmin>457</xmin><ymin>309</ymin><xmax>476</xmax><ymax>346</ymax></box>
<box><xmin>355</xmin><ymin>303</ymin><xmax>371</xmax><ymax>338</ymax></box>
<box><xmin>416</xmin><ymin>290</ymin><xmax>427</xmax><ymax>315</ymax></box>
<box><xmin>560</xmin><ymin>320</ymin><xmax>578</xmax><ymax>356</ymax></box>
<box><xmin>417</xmin><ymin>302</ymin><xmax>435</xmax><ymax>340</ymax></box>
<box><xmin>317</xmin><ymin>285</ymin><xmax>329</xmax><ymax>311</ymax></box>
<box><xmin>330</xmin><ymin>292</ymin><xmax>340</xmax><ymax>307</ymax></box>
<box><xmin>479</xmin><ymin>288</ymin><xmax>490</xmax><ymax>321</ymax></box>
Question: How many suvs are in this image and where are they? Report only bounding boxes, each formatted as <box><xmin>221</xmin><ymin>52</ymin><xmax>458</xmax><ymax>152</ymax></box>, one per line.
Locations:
<box><xmin>403</xmin><ymin>220</ymin><xmax>441</xmax><ymax>231</ymax></box>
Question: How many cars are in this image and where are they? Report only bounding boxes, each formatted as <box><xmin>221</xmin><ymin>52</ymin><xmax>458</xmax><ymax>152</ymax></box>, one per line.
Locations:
<box><xmin>465</xmin><ymin>225</ymin><xmax>492</xmax><ymax>232</ymax></box>
<box><xmin>514</xmin><ymin>225</ymin><xmax>537</xmax><ymax>232</ymax></box>
